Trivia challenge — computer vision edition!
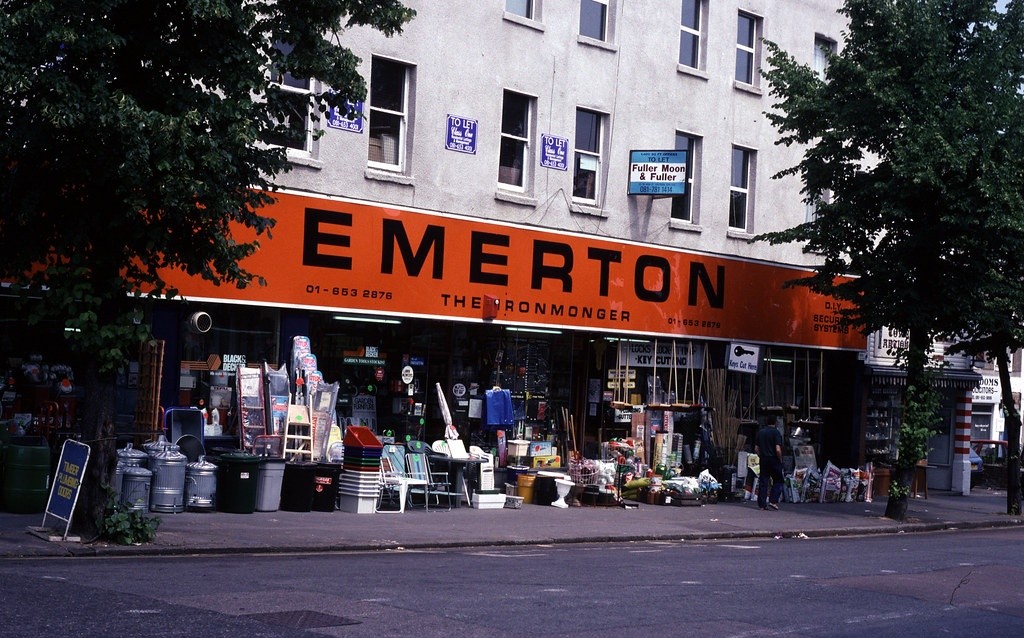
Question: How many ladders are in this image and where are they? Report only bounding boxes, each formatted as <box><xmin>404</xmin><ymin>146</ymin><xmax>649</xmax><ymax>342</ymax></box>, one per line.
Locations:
<box><xmin>234</xmin><ymin>336</ymin><xmax>340</xmax><ymax>460</ymax></box>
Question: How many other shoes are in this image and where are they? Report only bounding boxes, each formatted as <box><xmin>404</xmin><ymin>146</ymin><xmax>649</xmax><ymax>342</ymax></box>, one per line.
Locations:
<box><xmin>759</xmin><ymin>505</ymin><xmax>771</xmax><ymax>510</ymax></box>
<box><xmin>768</xmin><ymin>502</ymin><xmax>779</xmax><ymax>510</ymax></box>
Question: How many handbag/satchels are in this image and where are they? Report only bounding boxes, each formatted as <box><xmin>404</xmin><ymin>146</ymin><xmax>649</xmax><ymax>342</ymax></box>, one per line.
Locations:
<box><xmin>485</xmin><ymin>385</ymin><xmax>514</xmax><ymax>425</ymax></box>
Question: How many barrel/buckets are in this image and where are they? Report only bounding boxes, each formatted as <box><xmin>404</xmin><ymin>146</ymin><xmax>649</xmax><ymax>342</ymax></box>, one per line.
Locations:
<box><xmin>518</xmin><ymin>475</ymin><xmax>535</xmax><ymax>503</ymax></box>
<box><xmin>281</xmin><ymin>462</ymin><xmax>317</xmax><ymax>512</ymax></box>
<box><xmin>312</xmin><ymin>462</ymin><xmax>341</xmax><ymax>512</ymax></box>
<box><xmin>115</xmin><ymin>436</ymin><xmax>260</xmax><ymax>515</ymax></box>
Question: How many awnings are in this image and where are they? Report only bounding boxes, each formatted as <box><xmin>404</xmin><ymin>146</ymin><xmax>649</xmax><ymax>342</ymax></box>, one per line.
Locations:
<box><xmin>864</xmin><ymin>364</ymin><xmax>983</xmax><ymax>389</ymax></box>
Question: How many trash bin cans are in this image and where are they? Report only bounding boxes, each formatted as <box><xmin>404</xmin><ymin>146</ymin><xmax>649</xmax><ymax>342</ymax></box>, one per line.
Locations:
<box><xmin>254</xmin><ymin>455</ymin><xmax>287</xmax><ymax>512</ymax></box>
<box><xmin>214</xmin><ymin>448</ymin><xmax>261</xmax><ymax>514</ymax></box>
<box><xmin>120</xmin><ymin>464</ymin><xmax>153</xmax><ymax>514</ymax></box>
<box><xmin>142</xmin><ymin>436</ymin><xmax>180</xmax><ymax>470</ymax></box>
<box><xmin>281</xmin><ymin>460</ymin><xmax>319</xmax><ymax>513</ymax></box>
<box><xmin>150</xmin><ymin>446</ymin><xmax>188</xmax><ymax>514</ymax></box>
<box><xmin>186</xmin><ymin>455</ymin><xmax>218</xmax><ymax>511</ymax></box>
<box><xmin>109</xmin><ymin>442</ymin><xmax>149</xmax><ymax>503</ymax></box>
<box><xmin>311</xmin><ymin>461</ymin><xmax>342</xmax><ymax>513</ymax></box>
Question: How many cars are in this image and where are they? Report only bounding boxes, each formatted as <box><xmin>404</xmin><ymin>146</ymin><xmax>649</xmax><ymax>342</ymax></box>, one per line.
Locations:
<box><xmin>970</xmin><ymin>447</ymin><xmax>984</xmax><ymax>490</ymax></box>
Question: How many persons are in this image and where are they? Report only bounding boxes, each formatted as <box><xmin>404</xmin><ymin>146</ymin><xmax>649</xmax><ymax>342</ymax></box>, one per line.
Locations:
<box><xmin>755</xmin><ymin>414</ymin><xmax>784</xmax><ymax>511</ymax></box>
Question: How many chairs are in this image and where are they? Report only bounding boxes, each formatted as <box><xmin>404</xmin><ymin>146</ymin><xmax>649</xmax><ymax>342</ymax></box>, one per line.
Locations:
<box><xmin>380</xmin><ymin>445</ymin><xmax>430</xmax><ymax>513</ymax></box>
<box><xmin>407</xmin><ymin>440</ymin><xmax>448</xmax><ymax>466</ymax></box>
<box><xmin>405</xmin><ymin>453</ymin><xmax>452</xmax><ymax>511</ymax></box>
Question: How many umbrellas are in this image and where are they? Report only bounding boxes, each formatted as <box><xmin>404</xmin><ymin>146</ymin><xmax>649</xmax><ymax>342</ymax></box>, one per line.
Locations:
<box><xmin>436</xmin><ymin>382</ymin><xmax>470</xmax><ymax>506</ymax></box>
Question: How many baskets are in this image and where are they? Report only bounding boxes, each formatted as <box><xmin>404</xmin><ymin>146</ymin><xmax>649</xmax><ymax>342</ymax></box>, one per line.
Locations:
<box><xmin>568</xmin><ymin>462</ymin><xmax>599</xmax><ymax>486</ymax></box>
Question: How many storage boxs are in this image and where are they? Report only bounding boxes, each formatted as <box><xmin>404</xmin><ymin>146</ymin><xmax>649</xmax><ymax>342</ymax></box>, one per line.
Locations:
<box><xmin>166</xmin><ymin>409</ymin><xmax>203</xmax><ymax>446</ymax></box>
<box><xmin>508</xmin><ymin>444</ymin><xmax>529</xmax><ymax>457</ymax></box>
<box><xmin>471</xmin><ymin>492</ymin><xmax>507</xmax><ymax>510</ymax></box>
<box><xmin>505</xmin><ymin>496</ymin><xmax>524</xmax><ymax>509</ymax></box>
<box><xmin>338</xmin><ymin>425</ymin><xmax>383</xmax><ymax>514</ymax></box>
